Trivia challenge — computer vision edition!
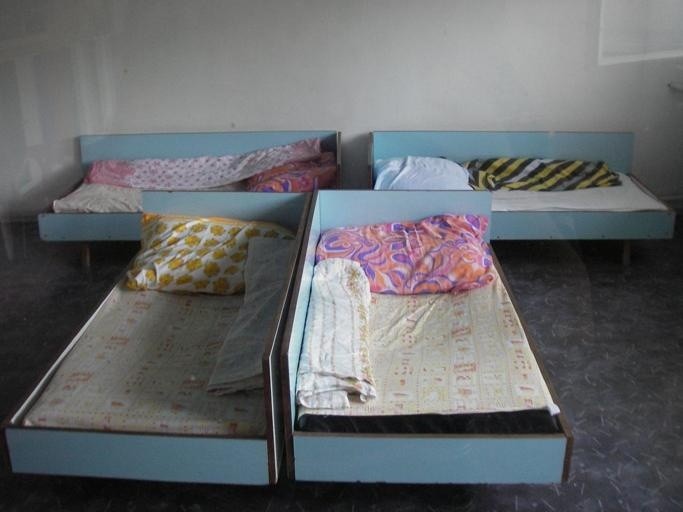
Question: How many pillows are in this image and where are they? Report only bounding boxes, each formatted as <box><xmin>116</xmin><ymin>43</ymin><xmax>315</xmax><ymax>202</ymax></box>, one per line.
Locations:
<box><xmin>126</xmin><ymin>214</ymin><xmax>297</xmax><ymax>296</ymax></box>
<box><xmin>315</xmin><ymin>213</ymin><xmax>494</xmax><ymax>296</ymax></box>
<box><xmin>243</xmin><ymin>150</ymin><xmax>337</xmax><ymax>192</ymax></box>
<box><xmin>372</xmin><ymin>155</ymin><xmax>474</xmax><ymax>191</ymax></box>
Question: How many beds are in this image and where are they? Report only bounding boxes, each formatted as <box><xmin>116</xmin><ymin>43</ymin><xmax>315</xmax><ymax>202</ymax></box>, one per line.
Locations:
<box><xmin>279</xmin><ymin>188</ymin><xmax>574</xmax><ymax>492</ymax></box>
<box><xmin>0</xmin><ymin>188</ymin><xmax>315</xmax><ymax>486</ymax></box>
<box><xmin>35</xmin><ymin>128</ymin><xmax>342</xmax><ymax>269</ymax></box>
<box><xmin>365</xmin><ymin>130</ymin><xmax>676</xmax><ymax>267</ymax></box>
